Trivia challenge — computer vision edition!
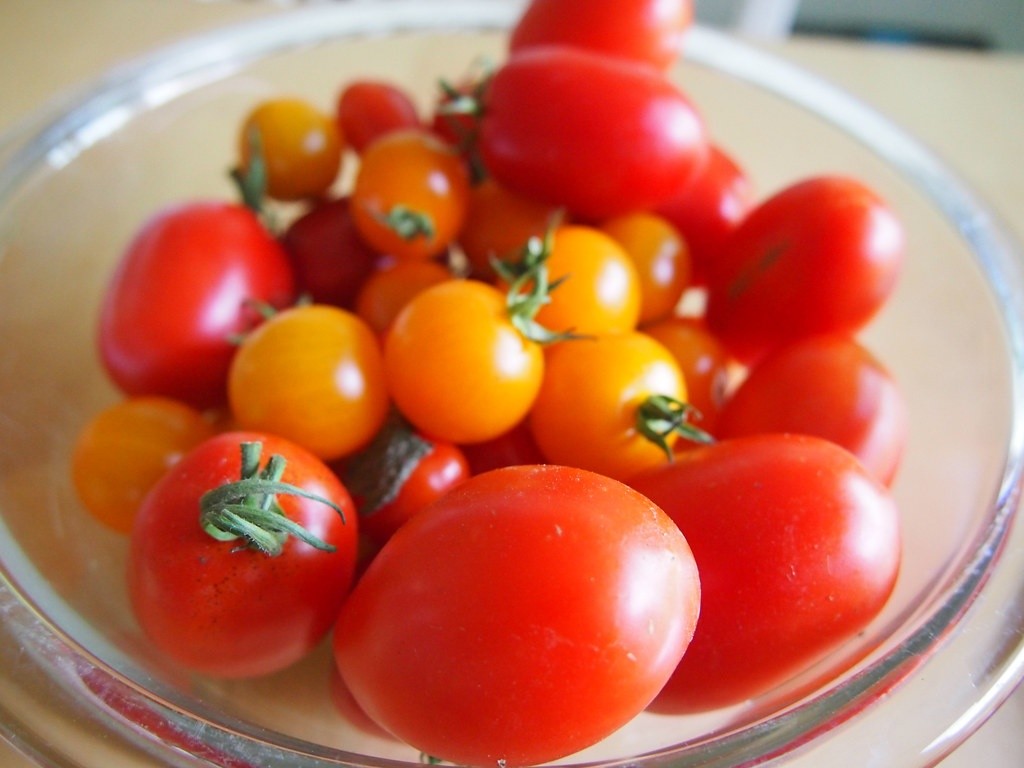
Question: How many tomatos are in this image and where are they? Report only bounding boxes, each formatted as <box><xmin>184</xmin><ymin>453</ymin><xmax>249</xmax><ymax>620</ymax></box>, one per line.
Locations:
<box><xmin>77</xmin><ymin>0</ymin><xmax>907</xmax><ymax>767</ymax></box>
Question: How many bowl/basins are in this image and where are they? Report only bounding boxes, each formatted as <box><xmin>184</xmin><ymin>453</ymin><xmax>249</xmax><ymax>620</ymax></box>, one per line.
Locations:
<box><xmin>0</xmin><ymin>0</ymin><xmax>1024</xmax><ymax>768</ymax></box>
